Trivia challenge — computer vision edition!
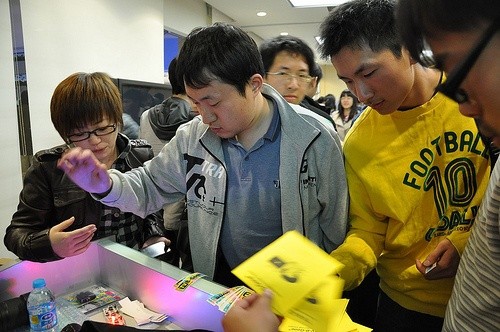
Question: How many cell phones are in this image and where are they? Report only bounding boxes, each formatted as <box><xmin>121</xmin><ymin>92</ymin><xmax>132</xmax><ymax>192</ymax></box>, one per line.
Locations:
<box><xmin>141</xmin><ymin>241</ymin><xmax>172</xmax><ymax>259</ymax></box>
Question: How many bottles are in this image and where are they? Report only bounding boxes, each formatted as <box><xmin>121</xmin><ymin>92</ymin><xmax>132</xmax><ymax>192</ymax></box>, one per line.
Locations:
<box><xmin>27</xmin><ymin>279</ymin><xmax>58</xmax><ymax>332</ymax></box>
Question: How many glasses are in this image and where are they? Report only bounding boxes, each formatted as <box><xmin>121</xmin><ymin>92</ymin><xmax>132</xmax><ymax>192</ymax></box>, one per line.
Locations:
<box><xmin>266</xmin><ymin>71</ymin><xmax>313</xmax><ymax>83</ymax></box>
<box><xmin>436</xmin><ymin>19</ymin><xmax>500</xmax><ymax>103</ymax></box>
<box><xmin>67</xmin><ymin>120</ymin><xmax>117</xmax><ymax>143</ymax></box>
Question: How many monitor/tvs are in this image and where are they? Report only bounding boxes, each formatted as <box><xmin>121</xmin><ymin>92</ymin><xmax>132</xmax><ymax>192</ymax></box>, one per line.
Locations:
<box><xmin>119</xmin><ymin>79</ymin><xmax>173</xmax><ymax>128</ymax></box>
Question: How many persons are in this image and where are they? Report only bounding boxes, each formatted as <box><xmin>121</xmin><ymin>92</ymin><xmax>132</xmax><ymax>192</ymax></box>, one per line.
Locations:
<box><xmin>58</xmin><ymin>23</ymin><xmax>348</xmax><ymax>293</ymax></box>
<box><xmin>4</xmin><ymin>72</ymin><xmax>171</xmax><ymax>263</ymax></box>
<box><xmin>121</xmin><ymin>85</ymin><xmax>164</xmax><ymax>140</ymax></box>
<box><xmin>222</xmin><ymin>0</ymin><xmax>500</xmax><ymax>332</ymax></box>
<box><xmin>141</xmin><ymin>58</ymin><xmax>193</xmax><ymax>273</ymax></box>
